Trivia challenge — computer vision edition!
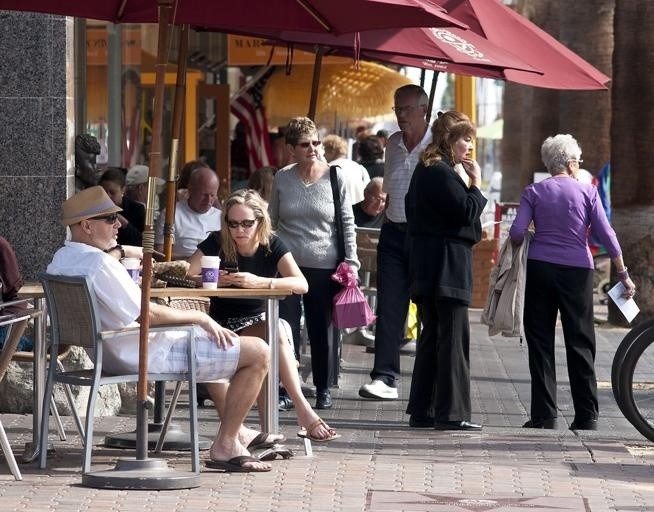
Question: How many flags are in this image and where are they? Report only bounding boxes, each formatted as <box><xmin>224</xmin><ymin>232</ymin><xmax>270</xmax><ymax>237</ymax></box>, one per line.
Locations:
<box><xmin>232</xmin><ymin>65</ymin><xmax>280</xmax><ymax>176</ymax></box>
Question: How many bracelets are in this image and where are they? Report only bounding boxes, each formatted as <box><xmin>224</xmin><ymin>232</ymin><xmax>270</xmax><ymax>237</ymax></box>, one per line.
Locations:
<box><xmin>615</xmin><ymin>267</ymin><xmax>631</xmax><ymax>282</ymax></box>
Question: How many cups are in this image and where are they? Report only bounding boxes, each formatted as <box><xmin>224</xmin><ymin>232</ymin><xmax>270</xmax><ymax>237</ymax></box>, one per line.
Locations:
<box><xmin>121</xmin><ymin>258</ymin><xmax>140</xmax><ymax>284</ymax></box>
<box><xmin>200</xmin><ymin>255</ymin><xmax>221</xmax><ymax>290</ymax></box>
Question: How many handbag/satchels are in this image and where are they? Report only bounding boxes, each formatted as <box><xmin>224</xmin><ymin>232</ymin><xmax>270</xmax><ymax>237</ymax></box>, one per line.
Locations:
<box><xmin>151</xmin><ymin>258</ymin><xmax>191</xmax><ymax>279</ymax></box>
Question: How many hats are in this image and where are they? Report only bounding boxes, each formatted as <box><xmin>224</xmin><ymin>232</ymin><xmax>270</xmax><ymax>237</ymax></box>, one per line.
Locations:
<box><xmin>61</xmin><ymin>184</ymin><xmax>124</xmax><ymax>226</ymax></box>
<box><xmin>127</xmin><ymin>166</ymin><xmax>166</xmax><ymax>185</ymax></box>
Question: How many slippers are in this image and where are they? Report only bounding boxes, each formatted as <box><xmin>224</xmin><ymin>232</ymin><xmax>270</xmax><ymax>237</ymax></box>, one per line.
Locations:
<box><xmin>245</xmin><ymin>430</ymin><xmax>287</xmax><ymax>453</ymax></box>
<box><xmin>206</xmin><ymin>456</ymin><xmax>272</xmax><ymax>473</ymax></box>
<box><xmin>297</xmin><ymin>419</ymin><xmax>342</xmax><ymax>442</ymax></box>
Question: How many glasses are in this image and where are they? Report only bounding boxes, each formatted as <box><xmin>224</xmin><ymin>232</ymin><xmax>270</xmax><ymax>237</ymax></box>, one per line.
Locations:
<box><xmin>371</xmin><ymin>196</ymin><xmax>390</xmax><ymax>205</ymax></box>
<box><xmin>568</xmin><ymin>159</ymin><xmax>583</xmax><ymax>165</ymax></box>
<box><xmin>91</xmin><ymin>215</ymin><xmax>118</xmax><ymax>223</ymax></box>
<box><xmin>297</xmin><ymin>141</ymin><xmax>322</xmax><ymax>149</ymax></box>
<box><xmin>225</xmin><ymin>218</ymin><xmax>257</xmax><ymax>228</ymax></box>
<box><xmin>392</xmin><ymin>104</ymin><xmax>425</xmax><ymax>113</ymax></box>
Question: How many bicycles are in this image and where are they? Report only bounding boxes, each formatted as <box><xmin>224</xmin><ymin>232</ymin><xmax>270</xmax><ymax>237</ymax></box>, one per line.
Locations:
<box><xmin>618</xmin><ymin>326</ymin><xmax>654</xmax><ymax>444</ymax></box>
<box><xmin>610</xmin><ymin>318</ymin><xmax>654</xmax><ymax>429</ymax></box>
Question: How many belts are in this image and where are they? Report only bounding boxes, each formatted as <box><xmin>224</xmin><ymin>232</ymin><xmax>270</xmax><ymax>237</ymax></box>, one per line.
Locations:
<box><xmin>384</xmin><ymin>217</ymin><xmax>408</xmax><ymax>230</ymax></box>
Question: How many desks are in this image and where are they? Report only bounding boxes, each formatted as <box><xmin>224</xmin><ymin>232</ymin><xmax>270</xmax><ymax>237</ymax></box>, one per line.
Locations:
<box><xmin>15</xmin><ymin>283</ymin><xmax>292</xmax><ymax>470</ymax></box>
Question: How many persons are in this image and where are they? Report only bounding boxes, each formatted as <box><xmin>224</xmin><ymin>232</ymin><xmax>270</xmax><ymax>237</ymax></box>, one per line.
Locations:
<box><xmin>95</xmin><ymin>120</ymin><xmax>400</xmax><ymax>227</ymax></box>
<box><xmin>264</xmin><ymin>112</ymin><xmax>363</xmax><ymax>410</ymax></box>
<box><xmin>508</xmin><ymin>131</ymin><xmax>639</xmax><ymax>434</ymax></box>
<box><xmin>151</xmin><ymin>166</ymin><xmax>229</xmax><ymax>262</ymax></box>
<box><xmin>0</xmin><ymin>230</ymin><xmax>33</xmax><ymax>376</ymax></box>
<box><xmin>41</xmin><ymin>183</ymin><xmax>287</xmax><ymax>474</ymax></box>
<box><xmin>358</xmin><ymin>82</ymin><xmax>478</xmax><ymax>406</ymax></box>
<box><xmin>183</xmin><ymin>188</ymin><xmax>346</xmax><ymax>446</ymax></box>
<box><xmin>404</xmin><ymin>108</ymin><xmax>490</xmax><ymax>433</ymax></box>
<box><xmin>92</xmin><ymin>169</ymin><xmax>148</xmax><ymax>245</ymax></box>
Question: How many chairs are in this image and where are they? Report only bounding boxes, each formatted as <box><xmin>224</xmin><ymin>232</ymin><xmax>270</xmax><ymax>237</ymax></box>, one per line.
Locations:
<box><xmin>34</xmin><ymin>271</ymin><xmax>201</xmax><ymax>477</ymax></box>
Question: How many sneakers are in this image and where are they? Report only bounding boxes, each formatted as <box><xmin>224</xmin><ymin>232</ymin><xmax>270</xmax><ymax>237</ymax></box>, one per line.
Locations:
<box><xmin>359</xmin><ymin>380</ymin><xmax>398</xmax><ymax>400</ymax></box>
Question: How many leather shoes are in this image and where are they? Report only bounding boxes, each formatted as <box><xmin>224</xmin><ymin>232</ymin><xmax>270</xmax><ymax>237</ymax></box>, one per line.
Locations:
<box><xmin>277</xmin><ymin>399</ymin><xmax>296</xmax><ymax>412</ymax></box>
<box><xmin>315</xmin><ymin>389</ymin><xmax>333</xmax><ymax>409</ymax></box>
<box><xmin>441</xmin><ymin>418</ymin><xmax>483</xmax><ymax>432</ymax></box>
<box><xmin>522</xmin><ymin>417</ymin><xmax>557</xmax><ymax>429</ymax></box>
<box><xmin>569</xmin><ymin>417</ymin><xmax>598</xmax><ymax>431</ymax></box>
<box><xmin>408</xmin><ymin>417</ymin><xmax>437</xmax><ymax>429</ymax></box>
